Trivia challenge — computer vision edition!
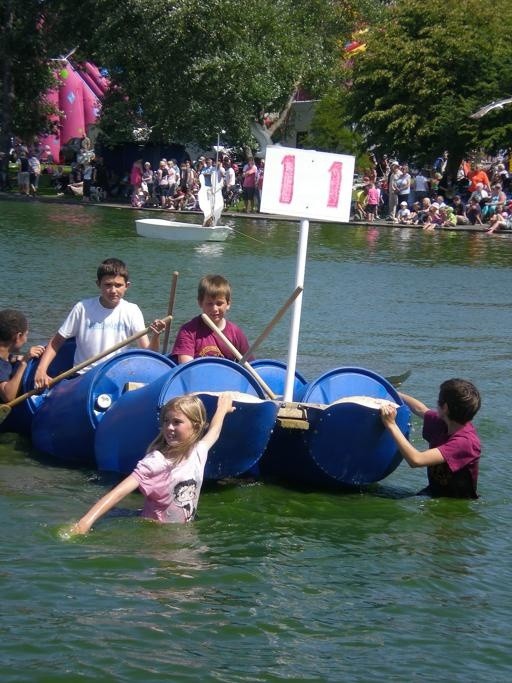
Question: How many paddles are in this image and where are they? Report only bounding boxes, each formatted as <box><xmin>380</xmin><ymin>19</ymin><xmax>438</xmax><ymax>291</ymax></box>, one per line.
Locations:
<box><xmin>387</xmin><ymin>370</ymin><xmax>413</xmax><ymax>388</ymax></box>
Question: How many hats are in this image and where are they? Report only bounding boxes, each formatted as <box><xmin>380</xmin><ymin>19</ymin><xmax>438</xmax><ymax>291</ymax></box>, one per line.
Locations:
<box><xmin>400</xmin><ymin>201</ymin><xmax>408</xmax><ymax>206</ymax></box>
<box><xmin>392</xmin><ymin>160</ymin><xmax>399</xmax><ymax>166</ymax></box>
<box><xmin>168</xmin><ymin>161</ymin><xmax>175</xmax><ymax>166</ymax></box>
<box><xmin>145</xmin><ymin>162</ymin><xmax>150</xmax><ymax>165</ymax></box>
<box><xmin>198</xmin><ymin>156</ymin><xmax>206</xmax><ymax>162</ymax></box>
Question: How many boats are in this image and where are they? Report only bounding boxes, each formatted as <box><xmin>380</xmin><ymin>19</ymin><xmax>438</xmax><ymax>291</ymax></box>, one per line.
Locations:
<box><xmin>0</xmin><ymin>339</ymin><xmax>411</xmax><ymax>487</ymax></box>
<box><xmin>135</xmin><ymin>218</ymin><xmax>232</xmax><ymax>242</ymax></box>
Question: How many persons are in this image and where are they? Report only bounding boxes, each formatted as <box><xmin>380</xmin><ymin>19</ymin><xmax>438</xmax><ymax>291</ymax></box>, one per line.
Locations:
<box><xmin>381</xmin><ymin>377</ymin><xmax>482</xmax><ymax>500</ymax></box>
<box><xmin>0</xmin><ymin>143</ymin><xmax>512</xmax><ymax>238</ymax></box>
<box><xmin>33</xmin><ymin>257</ymin><xmax>166</xmax><ymax>389</ymax></box>
<box><xmin>169</xmin><ymin>274</ymin><xmax>255</xmax><ymax>366</ymax></box>
<box><xmin>0</xmin><ymin>309</ymin><xmax>46</xmax><ymax>403</ymax></box>
<box><xmin>129</xmin><ymin>151</ymin><xmax>264</xmax><ymax>211</ymax></box>
<box><xmin>351</xmin><ymin>149</ymin><xmax>511</xmax><ymax>235</ymax></box>
<box><xmin>71</xmin><ymin>391</ymin><xmax>237</xmax><ymax>537</ymax></box>
<box><xmin>1</xmin><ymin>144</ymin><xmax>109</xmax><ymax>202</ymax></box>
